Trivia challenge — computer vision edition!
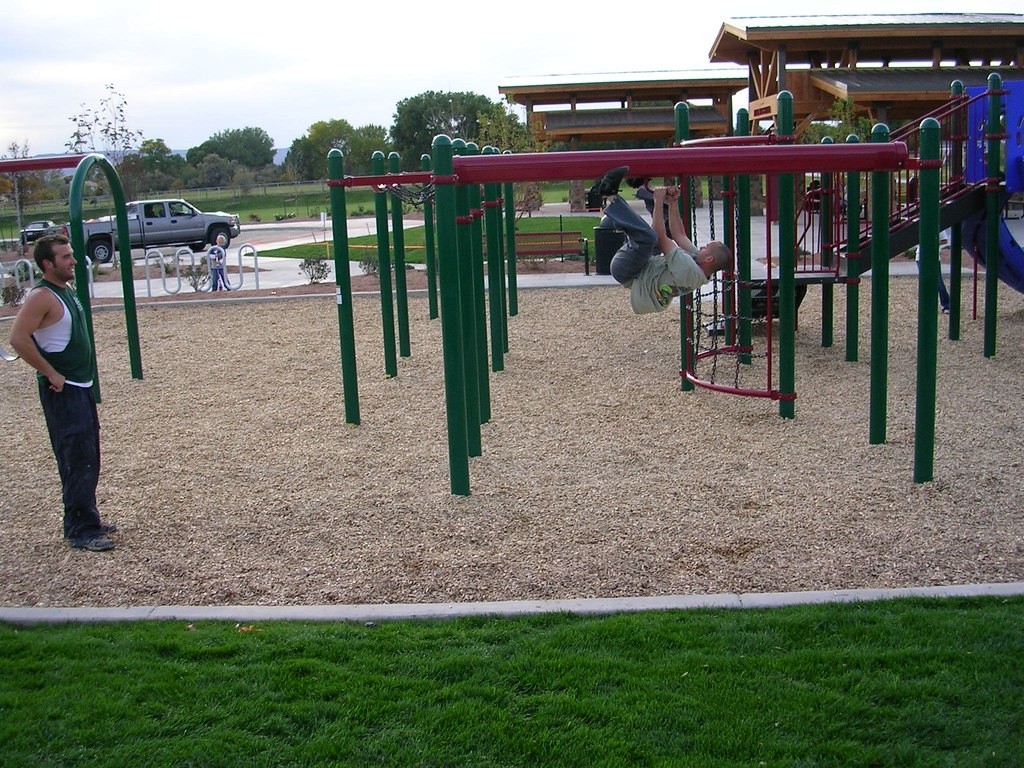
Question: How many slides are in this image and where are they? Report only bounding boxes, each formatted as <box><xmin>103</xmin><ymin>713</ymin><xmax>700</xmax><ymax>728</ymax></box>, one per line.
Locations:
<box><xmin>960</xmin><ymin>209</ymin><xmax>1024</xmax><ymax>292</ymax></box>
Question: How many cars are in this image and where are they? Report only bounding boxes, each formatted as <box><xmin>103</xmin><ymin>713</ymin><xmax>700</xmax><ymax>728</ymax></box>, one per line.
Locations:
<box><xmin>20</xmin><ymin>220</ymin><xmax>56</xmax><ymax>244</ymax></box>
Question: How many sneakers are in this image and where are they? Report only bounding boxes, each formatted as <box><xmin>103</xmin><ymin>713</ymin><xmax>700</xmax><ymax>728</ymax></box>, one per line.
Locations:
<box><xmin>69</xmin><ymin>536</ymin><xmax>116</xmax><ymax>551</ymax></box>
<box><xmin>626</xmin><ymin>176</ymin><xmax>653</xmax><ymax>189</ymax></box>
<box><xmin>597</xmin><ymin>166</ymin><xmax>629</xmax><ymax>197</ymax></box>
<box><xmin>100</xmin><ymin>520</ymin><xmax>117</xmax><ymax>533</ymax></box>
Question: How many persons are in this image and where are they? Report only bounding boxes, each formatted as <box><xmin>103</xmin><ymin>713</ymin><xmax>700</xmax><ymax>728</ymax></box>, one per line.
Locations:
<box><xmin>210</xmin><ymin>235</ymin><xmax>232</xmax><ymax>292</ymax></box>
<box><xmin>10</xmin><ymin>235</ymin><xmax>117</xmax><ymax>551</ymax></box>
<box><xmin>590</xmin><ymin>165</ymin><xmax>731</xmax><ymax>299</ymax></box>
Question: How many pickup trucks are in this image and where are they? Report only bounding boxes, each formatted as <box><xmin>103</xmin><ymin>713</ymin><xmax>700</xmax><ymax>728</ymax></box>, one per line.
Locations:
<box><xmin>46</xmin><ymin>199</ymin><xmax>241</xmax><ymax>265</ymax></box>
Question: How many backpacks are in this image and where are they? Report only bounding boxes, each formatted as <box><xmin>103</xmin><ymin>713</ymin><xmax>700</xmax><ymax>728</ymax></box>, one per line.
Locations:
<box><xmin>585</xmin><ymin>182</ymin><xmax>607</xmax><ymax>208</ymax></box>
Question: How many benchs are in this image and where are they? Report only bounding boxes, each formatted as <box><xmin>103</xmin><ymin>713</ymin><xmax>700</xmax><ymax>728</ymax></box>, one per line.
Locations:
<box><xmin>503</xmin><ymin>201</ymin><xmax>532</xmax><ymax>217</ymax></box>
<box><xmin>484</xmin><ymin>230</ymin><xmax>589</xmax><ymax>277</ymax></box>
